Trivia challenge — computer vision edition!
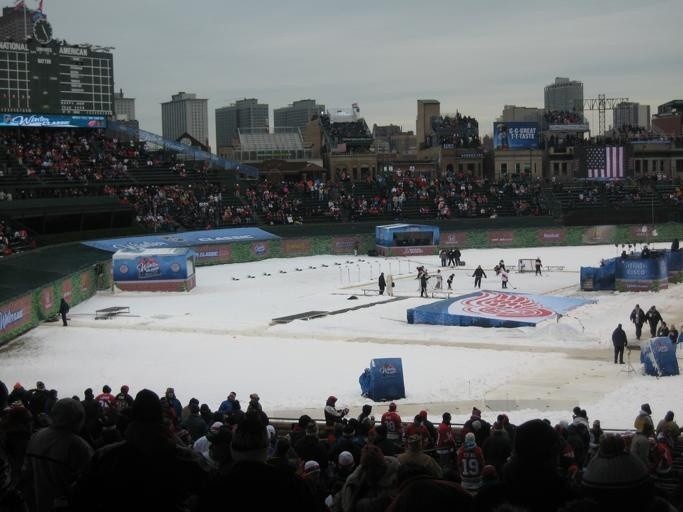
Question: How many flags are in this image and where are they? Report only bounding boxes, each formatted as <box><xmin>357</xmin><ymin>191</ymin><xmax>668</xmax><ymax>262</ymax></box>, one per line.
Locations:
<box><xmin>38</xmin><ymin>0</ymin><xmax>43</xmax><ymax>9</ymax></box>
<box><xmin>15</xmin><ymin>0</ymin><xmax>25</xmax><ymax>9</ymax></box>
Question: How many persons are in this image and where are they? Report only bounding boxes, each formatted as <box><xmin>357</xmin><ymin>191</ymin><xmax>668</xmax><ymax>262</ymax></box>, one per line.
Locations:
<box><xmin>472</xmin><ymin>265</ymin><xmax>485</xmax><ymax>288</ymax></box>
<box><xmin>255</xmin><ymin>162</ymin><xmax>682</xmax><ymax>227</ymax></box>
<box><xmin>501</xmin><ymin>273</ymin><xmax>508</xmax><ymax>288</ymax></box>
<box><xmin>385</xmin><ymin>274</ymin><xmax>394</xmax><ymax>295</ymax></box>
<box><xmin>417</xmin><ymin>265</ymin><xmax>455</xmax><ymax>297</ymax></box>
<box><xmin>0</xmin><ymin>124</ymin><xmax>261</xmax><ymax>259</ymax></box>
<box><xmin>630</xmin><ymin>304</ymin><xmax>679</xmax><ymax>344</ymax></box>
<box><xmin>379</xmin><ymin>272</ymin><xmax>386</xmax><ymax>295</ymax></box>
<box><xmin>360</xmin><ymin>369</ymin><xmax>370</xmax><ymax>394</ymax></box>
<box><xmin>612</xmin><ymin>324</ymin><xmax>627</xmax><ymax>363</ymax></box>
<box><xmin>621</xmin><ymin>238</ymin><xmax>681</xmax><ymax>259</ymax></box>
<box><xmin>60</xmin><ymin>298</ymin><xmax>69</xmax><ymax>326</ymax></box>
<box><xmin>499</xmin><ymin>260</ymin><xmax>506</xmax><ymax>271</ymax></box>
<box><xmin>536</xmin><ymin>258</ymin><xmax>542</xmax><ymax>275</ymax></box>
<box><xmin>437</xmin><ymin>245</ymin><xmax>463</xmax><ymax>265</ymax></box>
<box><xmin>1</xmin><ymin>380</ymin><xmax>683</xmax><ymax>511</ymax></box>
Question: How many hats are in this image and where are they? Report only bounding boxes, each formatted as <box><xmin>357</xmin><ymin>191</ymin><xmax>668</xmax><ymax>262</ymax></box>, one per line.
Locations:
<box><xmin>338</xmin><ymin>451</ymin><xmax>354</xmax><ymax>468</ymax></box>
<box><xmin>304</xmin><ymin>461</ymin><xmax>321</xmax><ymax>476</ymax></box>
<box><xmin>481</xmin><ymin>465</ymin><xmax>495</xmax><ymax>477</ymax></box>
<box><xmin>465</xmin><ymin>432</ymin><xmax>476</xmax><ymax>449</ymax></box>
